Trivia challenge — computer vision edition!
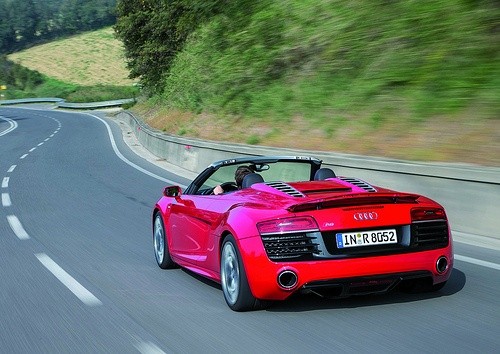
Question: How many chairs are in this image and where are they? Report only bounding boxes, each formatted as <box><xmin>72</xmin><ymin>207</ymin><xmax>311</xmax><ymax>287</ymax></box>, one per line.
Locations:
<box><xmin>242</xmin><ymin>173</ymin><xmax>263</xmax><ymax>189</ymax></box>
<box><xmin>313</xmin><ymin>167</ymin><xmax>335</xmax><ymax>181</ymax></box>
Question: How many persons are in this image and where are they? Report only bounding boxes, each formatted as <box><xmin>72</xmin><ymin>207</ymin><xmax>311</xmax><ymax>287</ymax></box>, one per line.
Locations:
<box><xmin>212</xmin><ymin>166</ymin><xmax>256</xmax><ymax>196</ymax></box>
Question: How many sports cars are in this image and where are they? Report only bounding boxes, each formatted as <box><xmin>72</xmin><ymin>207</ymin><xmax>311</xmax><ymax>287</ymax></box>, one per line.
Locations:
<box><xmin>152</xmin><ymin>154</ymin><xmax>455</xmax><ymax>312</ymax></box>
